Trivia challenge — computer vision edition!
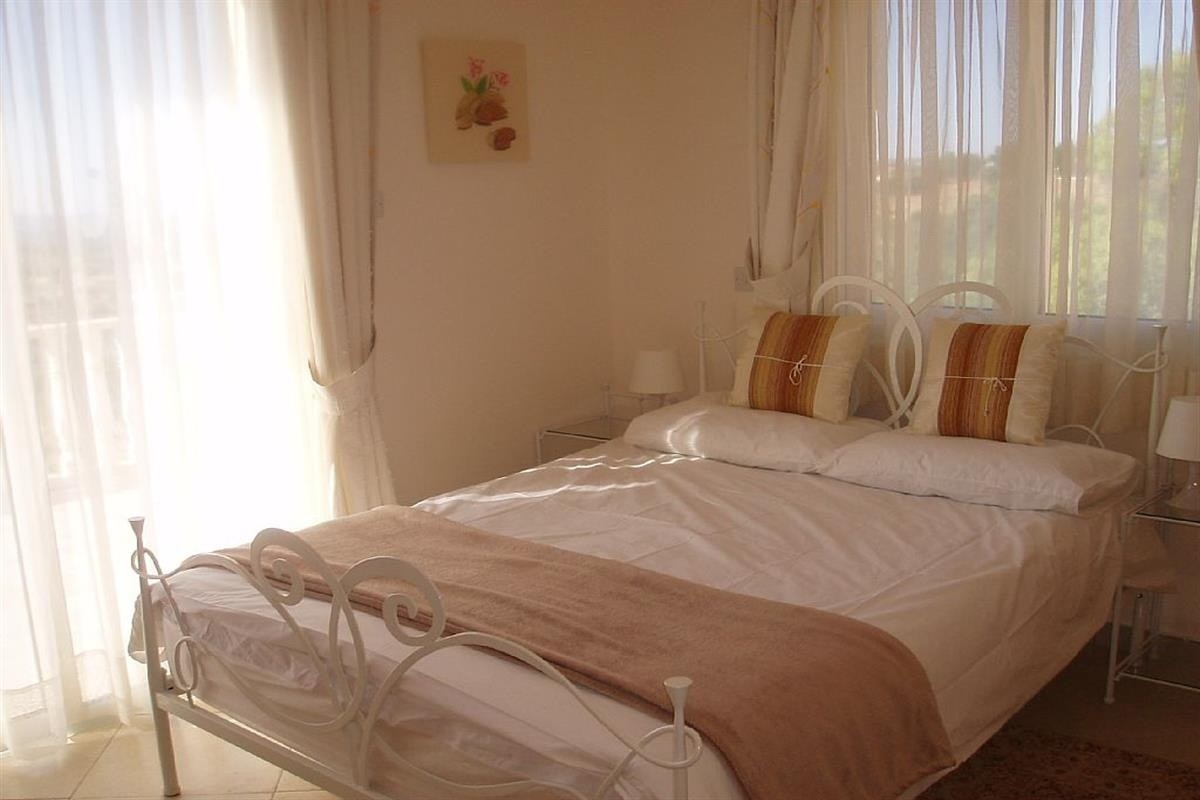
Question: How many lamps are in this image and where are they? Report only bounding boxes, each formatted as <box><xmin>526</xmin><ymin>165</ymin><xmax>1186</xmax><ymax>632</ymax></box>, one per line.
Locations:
<box><xmin>625</xmin><ymin>348</ymin><xmax>688</xmax><ymax>412</ymax></box>
<box><xmin>1156</xmin><ymin>396</ymin><xmax>1200</xmax><ymax>513</ymax></box>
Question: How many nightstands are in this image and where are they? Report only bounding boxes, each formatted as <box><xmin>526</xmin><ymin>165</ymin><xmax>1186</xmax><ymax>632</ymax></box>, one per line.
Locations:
<box><xmin>1105</xmin><ymin>484</ymin><xmax>1200</xmax><ymax>705</ymax></box>
<box><xmin>537</xmin><ymin>414</ymin><xmax>626</xmax><ymax>461</ymax></box>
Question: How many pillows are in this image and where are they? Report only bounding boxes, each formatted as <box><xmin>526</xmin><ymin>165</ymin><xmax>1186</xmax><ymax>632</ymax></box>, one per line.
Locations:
<box><xmin>822</xmin><ymin>423</ymin><xmax>1140</xmax><ymax>524</ymax></box>
<box><xmin>722</xmin><ymin>298</ymin><xmax>872</xmax><ymax>425</ymax></box>
<box><xmin>622</xmin><ymin>388</ymin><xmax>895</xmax><ymax>478</ymax></box>
<box><xmin>905</xmin><ymin>315</ymin><xmax>1068</xmax><ymax>443</ymax></box>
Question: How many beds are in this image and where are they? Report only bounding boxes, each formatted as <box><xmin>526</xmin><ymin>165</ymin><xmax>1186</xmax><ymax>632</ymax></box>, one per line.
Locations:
<box><xmin>126</xmin><ymin>276</ymin><xmax>1170</xmax><ymax>799</ymax></box>
